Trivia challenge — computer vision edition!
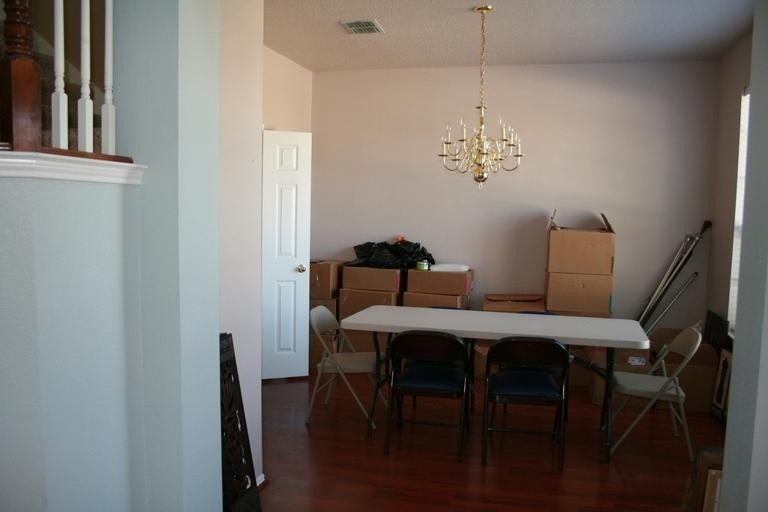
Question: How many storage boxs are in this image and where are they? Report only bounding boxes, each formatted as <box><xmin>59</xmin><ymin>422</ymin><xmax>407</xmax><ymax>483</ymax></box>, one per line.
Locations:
<box><xmin>544</xmin><ymin>270</ymin><xmax>614</xmax><ymax>314</ymax></box>
<box><xmin>547</xmin><ymin>208</ymin><xmax>616</xmax><ymax>275</ymax></box>
<box><xmin>616</xmin><ymin>349</ymin><xmax>650</xmax><ymax>375</ymax></box>
<box><xmin>339</xmin><ymin>332</ymin><xmax>388</xmax><ymax>353</ymax></box>
<box><xmin>403</xmin><ymin>292</ymin><xmax>471</xmax><ymax>310</ymax></box>
<box><xmin>566</xmin><ymin>346</ymin><xmax>601</xmax><ymax>387</ymax></box>
<box><xmin>310</xmin><ymin>297</ymin><xmax>339</xmax><ymax>317</ymax></box>
<box><xmin>339</xmin><ymin>289</ymin><xmax>397</xmax><ymax>331</ymax></box>
<box><xmin>310</xmin><ymin>260</ymin><xmax>349</xmax><ymax>299</ymax></box>
<box><xmin>342</xmin><ymin>265</ymin><xmax>401</xmax><ymax>293</ymax></box>
<box><xmin>310</xmin><ymin>335</ymin><xmax>338</xmax><ymax>376</ymax></box>
<box><xmin>407</xmin><ymin>268</ymin><xmax>473</xmax><ymax>296</ymax></box>
<box><xmin>482</xmin><ymin>293</ymin><xmax>545</xmax><ymax>312</ymax></box>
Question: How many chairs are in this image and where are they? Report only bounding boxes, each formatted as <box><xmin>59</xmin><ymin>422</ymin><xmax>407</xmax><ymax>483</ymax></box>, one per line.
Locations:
<box><xmin>481</xmin><ymin>336</ymin><xmax>570</xmax><ymax>471</ymax></box>
<box><xmin>304</xmin><ymin>306</ymin><xmax>390</xmax><ymax>431</ymax></box>
<box><xmin>383</xmin><ymin>330</ymin><xmax>474</xmax><ymax>465</ymax></box>
<box><xmin>601</xmin><ymin>327</ymin><xmax>703</xmax><ymax>463</ymax></box>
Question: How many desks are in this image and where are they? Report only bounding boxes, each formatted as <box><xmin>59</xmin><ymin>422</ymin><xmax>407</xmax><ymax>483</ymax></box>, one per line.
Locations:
<box><xmin>340</xmin><ymin>305</ymin><xmax>651</xmax><ymax>462</ymax></box>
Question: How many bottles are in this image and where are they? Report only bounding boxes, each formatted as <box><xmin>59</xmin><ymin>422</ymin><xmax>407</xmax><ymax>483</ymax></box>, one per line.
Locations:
<box><xmin>416</xmin><ymin>259</ymin><xmax>428</xmax><ymax>271</ymax></box>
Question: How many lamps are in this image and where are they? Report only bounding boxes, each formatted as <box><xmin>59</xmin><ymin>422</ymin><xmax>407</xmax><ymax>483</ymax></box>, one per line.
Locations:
<box><xmin>437</xmin><ymin>4</ymin><xmax>525</xmax><ymax>192</ymax></box>
